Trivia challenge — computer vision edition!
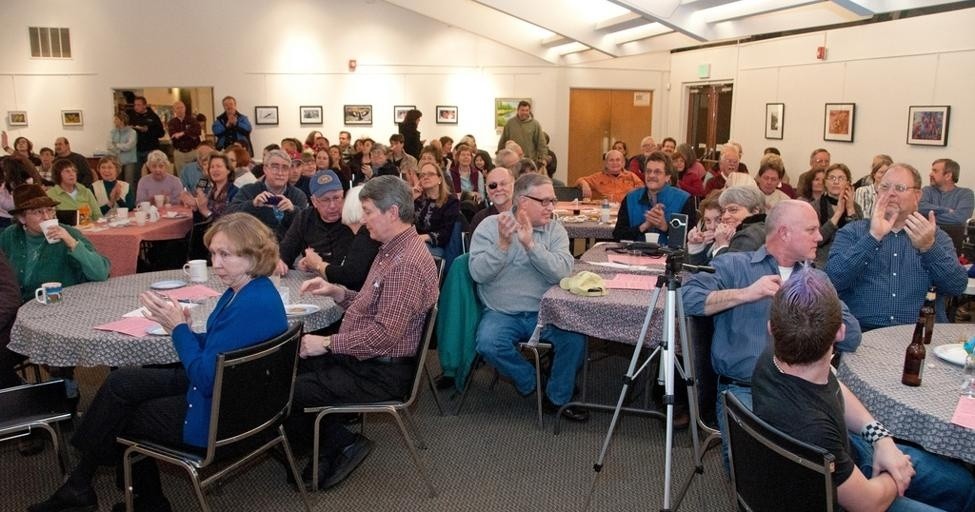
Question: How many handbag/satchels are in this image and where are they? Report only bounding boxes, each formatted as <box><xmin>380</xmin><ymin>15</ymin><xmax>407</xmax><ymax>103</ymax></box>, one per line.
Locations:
<box><xmin>625</xmin><ymin>241</ymin><xmax>664</xmax><ymax>257</ymax></box>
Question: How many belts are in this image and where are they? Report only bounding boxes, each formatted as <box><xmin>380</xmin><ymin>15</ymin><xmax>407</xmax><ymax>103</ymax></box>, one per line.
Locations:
<box><xmin>718</xmin><ymin>373</ymin><xmax>752</xmax><ymax>388</ymax></box>
<box><xmin>373</xmin><ymin>356</ymin><xmax>416</xmax><ymax>365</ymax></box>
<box><xmin>176</xmin><ymin>146</ymin><xmax>197</xmax><ymax>153</ymax></box>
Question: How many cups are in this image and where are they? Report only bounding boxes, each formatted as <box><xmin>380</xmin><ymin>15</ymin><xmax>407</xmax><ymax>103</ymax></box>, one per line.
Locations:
<box><xmin>267</xmin><ymin>272</ymin><xmax>281</xmax><ymax>292</ymax></box>
<box><xmin>117</xmin><ymin>194</ymin><xmax>165</xmax><ymax>228</ymax></box>
<box><xmin>39</xmin><ymin>219</ymin><xmax>63</xmax><ymax>245</ymax></box>
<box><xmin>34</xmin><ymin>281</ymin><xmax>64</xmax><ymax>306</ymax></box>
<box><xmin>182</xmin><ymin>259</ymin><xmax>209</xmax><ymax>282</ymax></box>
<box><xmin>644</xmin><ymin>233</ymin><xmax>660</xmax><ymax>246</ymax></box>
<box><xmin>627</xmin><ymin>251</ymin><xmax>641</xmax><ymax>269</ymax></box>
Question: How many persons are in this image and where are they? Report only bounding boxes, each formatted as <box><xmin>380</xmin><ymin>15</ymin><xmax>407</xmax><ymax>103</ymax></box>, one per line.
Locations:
<box><xmin>675</xmin><ymin>198</ymin><xmax>865</xmax><ymax>482</ymax></box>
<box><xmin>0</xmin><ymin>248</ymin><xmax>50</xmax><ymax>457</ymax></box>
<box><xmin>166</xmin><ymin>99</ymin><xmax>203</xmax><ymax>180</ymax></box>
<box><xmin>107</xmin><ymin>111</ymin><xmax>136</xmax><ymax>209</ymax></box>
<box><xmin>749</xmin><ymin>266</ymin><xmax>975</xmax><ymax>511</ymax></box>
<box><xmin>397</xmin><ymin>109</ymin><xmax>427</xmax><ymax>162</ymax></box>
<box><xmin>285</xmin><ymin>174</ymin><xmax>441</xmax><ymax>493</ymax></box>
<box><xmin>210</xmin><ymin>96</ymin><xmax>254</xmax><ymax>160</ymax></box>
<box><xmin>494</xmin><ymin>100</ymin><xmax>548</xmax><ymax>172</ymax></box>
<box><xmin>129</xmin><ymin>97</ymin><xmax>166</xmax><ymax>182</ymax></box>
<box><xmin>0</xmin><ymin>131</ymin><xmax>975</xmax><ymax>427</ymax></box>
<box><xmin>24</xmin><ymin>208</ymin><xmax>292</xmax><ymax>512</ymax></box>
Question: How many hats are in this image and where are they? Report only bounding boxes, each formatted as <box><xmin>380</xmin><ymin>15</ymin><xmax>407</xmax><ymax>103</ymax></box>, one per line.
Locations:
<box><xmin>308</xmin><ymin>169</ymin><xmax>343</xmax><ymax>197</ymax></box>
<box><xmin>7</xmin><ymin>184</ymin><xmax>60</xmax><ymax>215</ymax></box>
<box><xmin>286</xmin><ymin>151</ymin><xmax>304</xmax><ymax>165</ymax></box>
<box><xmin>559</xmin><ymin>270</ymin><xmax>607</xmax><ymax>297</ymax></box>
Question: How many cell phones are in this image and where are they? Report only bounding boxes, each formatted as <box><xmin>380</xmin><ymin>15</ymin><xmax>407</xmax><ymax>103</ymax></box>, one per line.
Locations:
<box><xmin>264</xmin><ymin>196</ymin><xmax>283</xmax><ymax>206</ymax></box>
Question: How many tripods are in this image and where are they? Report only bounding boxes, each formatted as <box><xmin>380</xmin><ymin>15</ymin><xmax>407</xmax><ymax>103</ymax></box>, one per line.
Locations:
<box><xmin>593</xmin><ymin>253</ymin><xmax>716</xmax><ymax>512</ymax></box>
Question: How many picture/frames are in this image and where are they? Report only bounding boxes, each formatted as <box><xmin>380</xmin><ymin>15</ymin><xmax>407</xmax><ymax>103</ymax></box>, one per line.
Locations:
<box><xmin>494</xmin><ymin>97</ymin><xmax>533</xmax><ymax>134</ymax></box>
<box><xmin>435</xmin><ymin>105</ymin><xmax>458</xmax><ymax>125</ymax></box>
<box><xmin>61</xmin><ymin>109</ymin><xmax>83</xmax><ymax>126</ymax></box>
<box><xmin>8</xmin><ymin>110</ymin><xmax>29</xmax><ymax>126</ymax></box>
<box><xmin>823</xmin><ymin>102</ymin><xmax>856</xmax><ymax>144</ymax></box>
<box><xmin>393</xmin><ymin>105</ymin><xmax>417</xmax><ymax>125</ymax></box>
<box><xmin>254</xmin><ymin>105</ymin><xmax>280</xmax><ymax>126</ymax></box>
<box><xmin>343</xmin><ymin>105</ymin><xmax>373</xmax><ymax>126</ymax></box>
<box><xmin>299</xmin><ymin>105</ymin><xmax>322</xmax><ymax>125</ymax></box>
<box><xmin>906</xmin><ymin>104</ymin><xmax>952</xmax><ymax>147</ymax></box>
<box><xmin>764</xmin><ymin>102</ymin><xmax>786</xmax><ymax>142</ymax></box>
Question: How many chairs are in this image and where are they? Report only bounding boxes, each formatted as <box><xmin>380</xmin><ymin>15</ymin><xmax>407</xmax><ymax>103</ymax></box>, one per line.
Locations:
<box><xmin>14</xmin><ymin>349</ymin><xmax>42</xmax><ymax>385</ymax></box>
<box><xmin>0</xmin><ymin>377</ymin><xmax>74</xmax><ymax>484</ymax></box>
<box><xmin>668</xmin><ymin>314</ymin><xmax>722</xmax><ymax>511</ymax></box>
<box><xmin>722</xmin><ymin>389</ymin><xmax>839</xmax><ymax>511</ymax></box>
<box><xmin>115</xmin><ymin>321</ymin><xmax>313</xmax><ymax>512</ymax></box>
<box><xmin>461</xmin><ymin>231</ymin><xmax>470</xmax><ymax>254</ymax></box>
<box><xmin>303</xmin><ymin>255</ymin><xmax>446</xmax><ymax>498</ymax></box>
<box><xmin>452</xmin><ymin>253</ymin><xmax>553</xmax><ymax>433</ymax></box>
<box><xmin>91</xmin><ymin>168</ymin><xmax>99</xmax><ymax>183</ymax></box>
<box><xmin>552</xmin><ymin>185</ymin><xmax>582</xmax><ymax>202</ymax></box>
<box><xmin>161</xmin><ymin>218</ymin><xmax>212</xmax><ymax>270</ymax></box>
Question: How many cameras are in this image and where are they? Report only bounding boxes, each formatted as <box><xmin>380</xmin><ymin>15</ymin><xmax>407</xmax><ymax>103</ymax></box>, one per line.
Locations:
<box><xmin>668</xmin><ymin>212</ymin><xmax>688</xmax><ymax>249</ymax></box>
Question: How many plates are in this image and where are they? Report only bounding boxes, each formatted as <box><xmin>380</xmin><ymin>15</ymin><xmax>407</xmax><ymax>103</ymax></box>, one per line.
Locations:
<box><xmin>147</xmin><ymin>326</ymin><xmax>172</xmax><ymax>335</ymax></box>
<box><xmin>281</xmin><ymin>304</ymin><xmax>320</xmax><ymax>317</ymax></box>
<box><xmin>933</xmin><ymin>344</ymin><xmax>970</xmax><ymax>364</ymax></box>
<box><xmin>162</xmin><ymin>214</ymin><xmax>188</xmax><ymax>220</ymax></box>
<box><xmin>148</xmin><ymin>280</ymin><xmax>185</xmax><ymax>289</ymax></box>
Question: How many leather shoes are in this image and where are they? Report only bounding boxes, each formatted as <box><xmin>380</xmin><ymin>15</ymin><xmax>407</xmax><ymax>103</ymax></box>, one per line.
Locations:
<box><xmin>302</xmin><ymin>432</ymin><xmax>375</xmax><ymax>492</ymax></box>
<box><xmin>26</xmin><ymin>484</ymin><xmax>98</xmax><ymax>512</ymax></box>
<box><xmin>433</xmin><ymin>370</ymin><xmax>469</xmax><ymax>392</ymax></box>
<box><xmin>110</xmin><ymin>496</ymin><xmax>171</xmax><ymax>511</ymax></box>
<box><xmin>540</xmin><ymin>392</ymin><xmax>590</xmax><ymax>423</ymax></box>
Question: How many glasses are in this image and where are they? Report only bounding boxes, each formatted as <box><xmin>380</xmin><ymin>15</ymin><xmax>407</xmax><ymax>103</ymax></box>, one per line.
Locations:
<box><xmin>525</xmin><ymin>196</ymin><xmax>558</xmax><ymax>206</ymax></box>
<box><xmin>878</xmin><ymin>182</ymin><xmax>919</xmax><ymax>193</ymax></box>
<box><xmin>827</xmin><ymin>175</ymin><xmax>848</xmax><ymax>183</ymax></box>
<box><xmin>720</xmin><ymin>206</ymin><xmax>750</xmax><ymax>214</ymax></box>
<box><xmin>813</xmin><ymin>159</ymin><xmax>830</xmax><ymax>164</ymax></box>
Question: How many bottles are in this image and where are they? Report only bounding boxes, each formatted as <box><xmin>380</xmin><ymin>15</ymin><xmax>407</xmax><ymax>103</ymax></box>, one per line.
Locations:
<box><xmin>602</xmin><ymin>196</ymin><xmax>610</xmax><ymax>225</ymax></box>
<box><xmin>901</xmin><ymin>281</ymin><xmax>937</xmax><ymax>387</ymax></box>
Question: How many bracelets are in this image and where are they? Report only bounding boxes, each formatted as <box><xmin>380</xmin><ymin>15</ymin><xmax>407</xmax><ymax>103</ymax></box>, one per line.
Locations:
<box><xmin>860</xmin><ymin>420</ymin><xmax>896</xmax><ymax>448</ymax></box>
